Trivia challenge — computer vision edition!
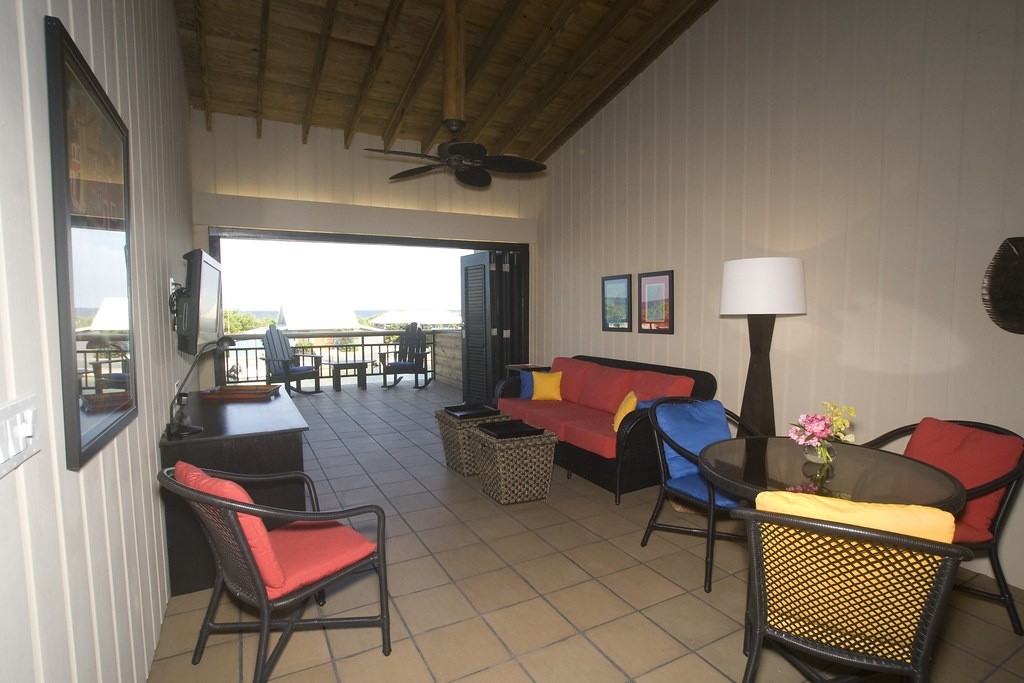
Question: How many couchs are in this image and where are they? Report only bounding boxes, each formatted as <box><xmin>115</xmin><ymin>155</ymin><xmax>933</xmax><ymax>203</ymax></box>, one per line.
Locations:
<box><xmin>495</xmin><ymin>354</ymin><xmax>716</xmax><ymax>505</ymax></box>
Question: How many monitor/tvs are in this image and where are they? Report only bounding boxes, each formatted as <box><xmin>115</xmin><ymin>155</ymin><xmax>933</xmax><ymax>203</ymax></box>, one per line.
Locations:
<box><xmin>174</xmin><ymin>250</ymin><xmax>222</xmax><ymax>356</ymax></box>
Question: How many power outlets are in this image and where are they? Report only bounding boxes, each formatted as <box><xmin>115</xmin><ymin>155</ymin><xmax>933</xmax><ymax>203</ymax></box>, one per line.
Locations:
<box><xmin>174</xmin><ymin>380</ymin><xmax>182</xmax><ymax>403</ymax></box>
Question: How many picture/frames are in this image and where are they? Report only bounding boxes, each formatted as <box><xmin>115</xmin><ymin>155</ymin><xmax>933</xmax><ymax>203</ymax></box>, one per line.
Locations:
<box><xmin>601</xmin><ymin>273</ymin><xmax>632</xmax><ymax>332</ymax></box>
<box><xmin>638</xmin><ymin>270</ymin><xmax>674</xmax><ymax>333</ymax></box>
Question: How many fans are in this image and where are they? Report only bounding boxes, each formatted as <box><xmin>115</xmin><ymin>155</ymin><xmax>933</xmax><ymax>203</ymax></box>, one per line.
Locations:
<box><xmin>363</xmin><ymin>0</ymin><xmax>547</xmax><ymax>188</ymax></box>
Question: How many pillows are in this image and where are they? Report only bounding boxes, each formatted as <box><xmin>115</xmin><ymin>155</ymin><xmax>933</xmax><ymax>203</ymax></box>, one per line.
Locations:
<box><xmin>517</xmin><ymin>370</ymin><xmax>534</xmax><ymax>398</ymax></box>
<box><xmin>655</xmin><ymin>401</ymin><xmax>735</xmax><ymax>476</ymax></box>
<box><xmin>901</xmin><ymin>416</ymin><xmax>1024</xmax><ymax>530</ymax></box>
<box><xmin>531</xmin><ymin>371</ymin><xmax>563</xmax><ymax>402</ymax></box>
<box><xmin>172</xmin><ymin>461</ymin><xmax>284</xmax><ymax>588</ymax></box>
<box><xmin>757</xmin><ymin>490</ymin><xmax>954</xmax><ymax>646</ymax></box>
<box><xmin>614</xmin><ymin>391</ymin><xmax>636</xmax><ymax>432</ymax></box>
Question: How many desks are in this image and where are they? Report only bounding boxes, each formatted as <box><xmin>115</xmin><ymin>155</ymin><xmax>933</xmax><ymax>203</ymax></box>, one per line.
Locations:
<box><xmin>159</xmin><ymin>385</ymin><xmax>306</xmax><ymax>596</ymax></box>
<box><xmin>321</xmin><ymin>359</ymin><xmax>376</xmax><ymax>393</ymax></box>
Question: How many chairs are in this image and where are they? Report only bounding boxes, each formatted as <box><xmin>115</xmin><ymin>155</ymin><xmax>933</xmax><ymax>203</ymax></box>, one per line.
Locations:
<box><xmin>380</xmin><ymin>323</ymin><xmax>434</xmax><ymax>389</ymax></box>
<box><xmin>639</xmin><ymin>397</ymin><xmax>769</xmax><ymax>591</ymax></box>
<box><xmin>742</xmin><ymin>491</ymin><xmax>972</xmax><ymax>683</ymax></box>
<box><xmin>262</xmin><ymin>324</ymin><xmax>323</xmax><ymax>398</ymax></box>
<box><xmin>89</xmin><ymin>359</ymin><xmax>130</xmax><ymax>394</ymax></box>
<box><xmin>858</xmin><ymin>419</ymin><xmax>1024</xmax><ymax>638</ymax></box>
<box><xmin>159</xmin><ymin>465</ymin><xmax>392</xmax><ymax>683</ymax></box>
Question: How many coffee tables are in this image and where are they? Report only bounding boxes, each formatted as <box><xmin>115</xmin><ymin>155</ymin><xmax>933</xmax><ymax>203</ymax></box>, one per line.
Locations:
<box><xmin>435</xmin><ymin>404</ymin><xmax>500</xmax><ymax>475</ymax></box>
<box><xmin>467</xmin><ymin>419</ymin><xmax>558</xmax><ymax>504</ymax></box>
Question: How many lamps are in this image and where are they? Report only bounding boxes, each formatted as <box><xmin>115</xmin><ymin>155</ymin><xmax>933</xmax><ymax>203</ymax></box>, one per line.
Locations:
<box><xmin>718</xmin><ymin>258</ymin><xmax>809</xmax><ymax>437</ymax></box>
<box><xmin>166</xmin><ymin>337</ymin><xmax>235</xmax><ymax>436</ymax></box>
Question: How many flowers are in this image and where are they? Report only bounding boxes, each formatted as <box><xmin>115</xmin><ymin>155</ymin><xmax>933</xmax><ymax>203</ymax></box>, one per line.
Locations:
<box><xmin>787</xmin><ymin>404</ymin><xmax>857</xmax><ymax>463</ymax></box>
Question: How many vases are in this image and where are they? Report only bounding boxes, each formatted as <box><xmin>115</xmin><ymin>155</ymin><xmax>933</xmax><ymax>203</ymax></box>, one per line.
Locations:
<box><xmin>802</xmin><ymin>444</ymin><xmax>836</xmax><ymax>478</ymax></box>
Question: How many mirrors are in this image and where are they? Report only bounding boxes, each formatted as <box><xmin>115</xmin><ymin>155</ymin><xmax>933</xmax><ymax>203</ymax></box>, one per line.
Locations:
<box><xmin>46</xmin><ymin>17</ymin><xmax>139</xmax><ymax>473</ymax></box>
